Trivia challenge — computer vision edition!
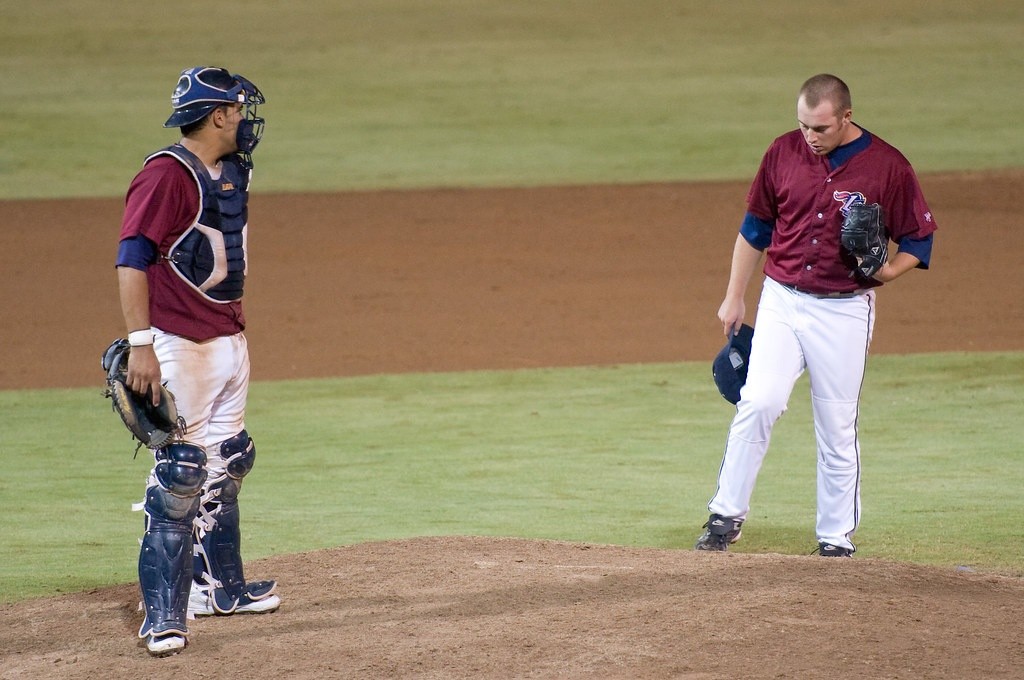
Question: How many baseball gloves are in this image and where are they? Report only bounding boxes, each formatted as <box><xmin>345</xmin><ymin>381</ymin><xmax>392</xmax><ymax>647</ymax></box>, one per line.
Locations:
<box><xmin>840</xmin><ymin>204</ymin><xmax>890</xmax><ymax>281</ymax></box>
<box><xmin>100</xmin><ymin>370</ymin><xmax>186</xmax><ymax>459</ymax></box>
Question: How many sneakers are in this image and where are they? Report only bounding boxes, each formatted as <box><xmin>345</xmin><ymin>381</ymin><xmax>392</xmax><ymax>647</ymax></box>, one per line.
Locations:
<box><xmin>145</xmin><ymin>633</ymin><xmax>185</xmax><ymax>654</ymax></box>
<box><xmin>811</xmin><ymin>542</ymin><xmax>852</xmax><ymax>559</ymax></box>
<box><xmin>188</xmin><ymin>579</ymin><xmax>280</xmax><ymax>614</ymax></box>
<box><xmin>695</xmin><ymin>514</ymin><xmax>742</xmax><ymax>554</ymax></box>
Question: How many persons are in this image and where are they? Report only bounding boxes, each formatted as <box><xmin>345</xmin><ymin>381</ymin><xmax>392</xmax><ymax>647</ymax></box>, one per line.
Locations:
<box><xmin>695</xmin><ymin>73</ymin><xmax>937</xmax><ymax>558</ymax></box>
<box><xmin>116</xmin><ymin>66</ymin><xmax>279</xmax><ymax>657</ymax></box>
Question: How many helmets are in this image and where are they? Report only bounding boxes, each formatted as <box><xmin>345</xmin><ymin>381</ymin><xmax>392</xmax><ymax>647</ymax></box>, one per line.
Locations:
<box><xmin>163</xmin><ymin>65</ymin><xmax>242</xmax><ymax>126</ymax></box>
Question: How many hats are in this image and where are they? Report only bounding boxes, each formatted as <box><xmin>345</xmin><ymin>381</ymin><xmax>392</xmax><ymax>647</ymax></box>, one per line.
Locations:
<box><xmin>713</xmin><ymin>322</ymin><xmax>755</xmax><ymax>406</ymax></box>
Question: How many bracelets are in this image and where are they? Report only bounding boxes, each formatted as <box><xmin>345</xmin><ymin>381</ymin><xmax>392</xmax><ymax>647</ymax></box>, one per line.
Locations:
<box><xmin>128</xmin><ymin>328</ymin><xmax>155</xmax><ymax>347</ymax></box>
